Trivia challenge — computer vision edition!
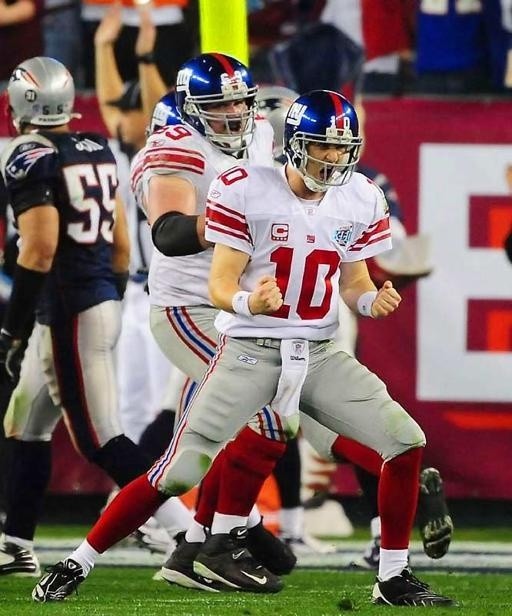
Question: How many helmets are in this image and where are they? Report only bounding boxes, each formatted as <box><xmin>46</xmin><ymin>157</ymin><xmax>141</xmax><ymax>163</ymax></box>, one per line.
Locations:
<box><xmin>143</xmin><ymin>52</ymin><xmax>364</xmax><ymax>192</ymax></box>
<box><xmin>5</xmin><ymin>57</ymin><xmax>82</xmax><ymax>128</ymax></box>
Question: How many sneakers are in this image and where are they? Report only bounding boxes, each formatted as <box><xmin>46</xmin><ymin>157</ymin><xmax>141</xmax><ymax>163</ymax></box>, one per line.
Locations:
<box><xmin>161</xmin><ymin>515</ymin><xmax>297</xmax><ymax>593</ymax></box>
<box><xmin>347</xmin><ymin>534</ymin><xmax>413</xmax><ymax>571</ymax></box>
<box><xmin>0</xmin><ymin>533</ymin><xmax>41</xmax><ymax>579</ymax></box>
<box><xmin>416</xmin><ymin>466</ymin><xmax>454</xmax><ymax>560</ymax></box>
<box><xmin>371</xmin><ymin>566</ymin><xmax>454</xmax><ymax>608</ymax></box>
<box><xmin>100</xmin><ymin>487</ymin><xmax>170</xmax><ymax>555</ymax></box>
<box><xmin>31</xmin><ymin>555</ymin><xmax>88</xmax><ymax>604</ymax></box>
<box><xmin>276</xmin><ymin>526</ymin><xmax>336</xmax><ymax>556</ymax></box>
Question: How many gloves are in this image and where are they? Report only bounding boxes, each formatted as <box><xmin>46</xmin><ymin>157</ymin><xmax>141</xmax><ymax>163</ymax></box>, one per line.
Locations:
<box><xmin>1</xmin><ymin>331</ymin><xmax>21</xmax><ymax>381</ymax></box>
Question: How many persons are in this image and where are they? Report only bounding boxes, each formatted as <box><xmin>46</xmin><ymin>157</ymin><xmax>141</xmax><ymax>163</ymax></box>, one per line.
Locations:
<box><xmin>92</xmin><ymin>0</ymin><xmax>455</xmax><ymax>596</ymax></box>
<box><xmin>0</xmin><ymin>54</ymin><xmax>191</xmax><ymax>576</ymax></box>
<box><xmin>31</xmin><ymin>88</ymin><xmax>457</xmax><ymax>607</ymax></box>
<box><xmin>0</xmin><ymin>0</ymin><xmax>509</xmax><ymax>96</ymax></box>
